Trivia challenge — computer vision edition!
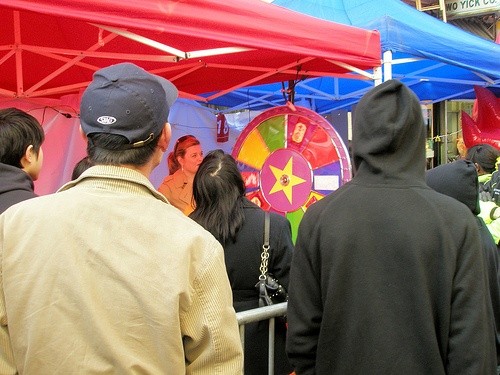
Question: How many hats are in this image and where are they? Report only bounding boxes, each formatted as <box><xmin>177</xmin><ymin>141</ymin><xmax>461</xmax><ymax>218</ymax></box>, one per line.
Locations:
<box><xmin>80</xmin><ymin>63</ymin><xmax>178</xmax><ymax>150</ymax></box>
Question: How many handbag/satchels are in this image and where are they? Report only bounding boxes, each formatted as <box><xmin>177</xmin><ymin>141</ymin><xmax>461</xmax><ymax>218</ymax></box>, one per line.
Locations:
<box><xmin>255</xmin><ymin>212</ymin><xmax>289</xmax><ymax>330</ymax></box>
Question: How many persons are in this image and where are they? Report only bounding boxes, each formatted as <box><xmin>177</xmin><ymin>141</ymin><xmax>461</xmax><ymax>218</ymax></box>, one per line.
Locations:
<box><xmin>0</xmin><ymin>108</ymin><xmax>93</xmax><ymax>213</ymax></box>
<box><xmin>0</xmin><ymin>62</ymin><xmax>244</xmax><ymax>375</ymax></box>
<box><xmin>285</xmin><ymin>80</ymin><xmax>500</xmax><ymax>375</ymax></box>
<box><xmin>189</xmin><ymin>149</ymin><xmax>293</xmax><ymax>375</ymax></box>
<box><xmin>157</xmin><ymin>135</ymin><xmax>203</xmax><ymax>216</ymax></box>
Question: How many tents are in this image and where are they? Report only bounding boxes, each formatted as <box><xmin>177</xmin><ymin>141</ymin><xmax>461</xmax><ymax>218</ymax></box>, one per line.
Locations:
<box><xmin>0</xmin><ymin>0</ymin><xmax>499</xmax><ymax>151</ymax></box>
